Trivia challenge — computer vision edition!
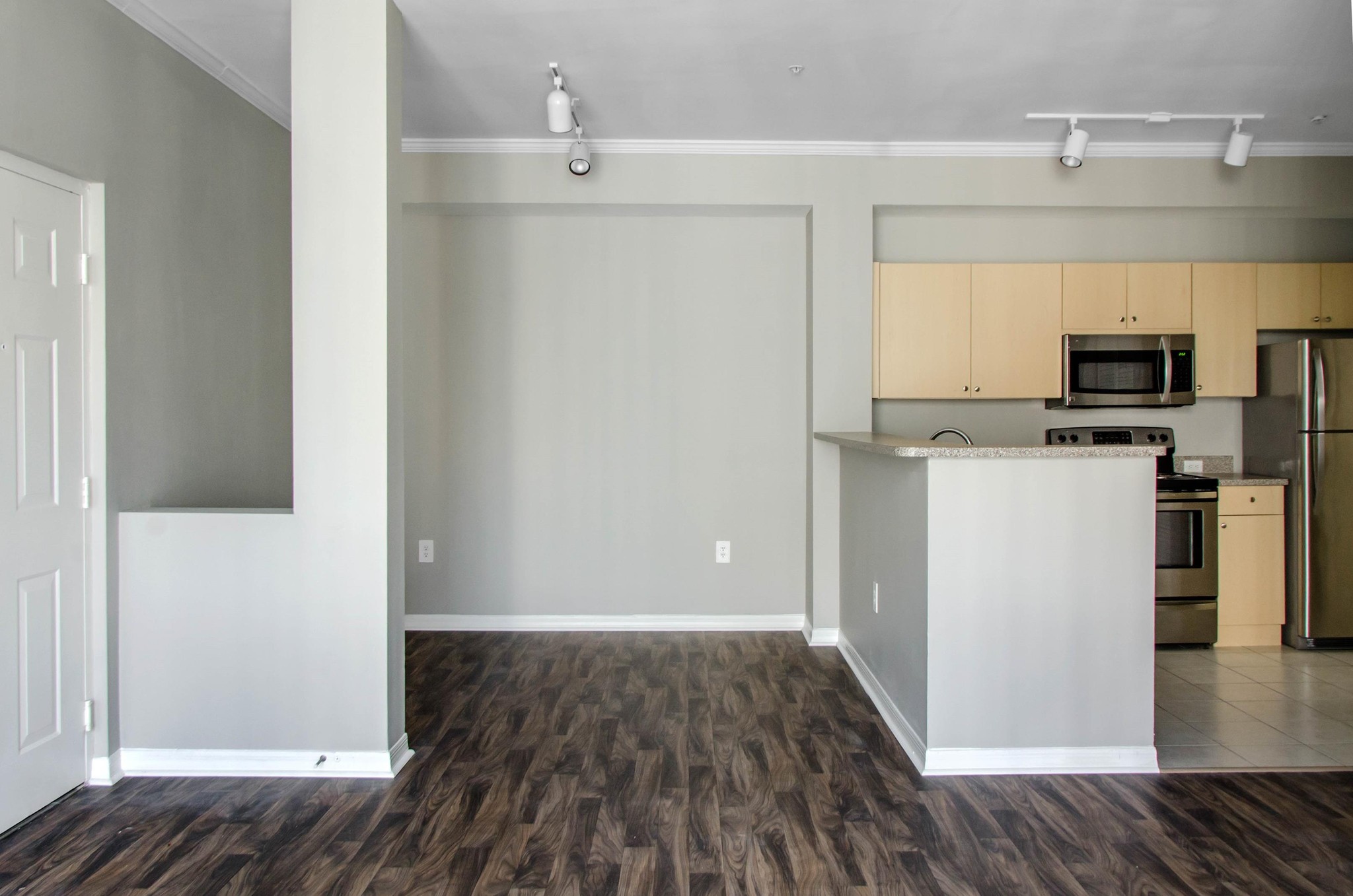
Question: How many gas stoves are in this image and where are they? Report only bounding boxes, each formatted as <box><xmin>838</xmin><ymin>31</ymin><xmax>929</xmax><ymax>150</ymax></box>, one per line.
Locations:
<box><xmin>1048</xmin><ymin>425</ymin><xmax>1219</xmax><ymax>499</ymax></box>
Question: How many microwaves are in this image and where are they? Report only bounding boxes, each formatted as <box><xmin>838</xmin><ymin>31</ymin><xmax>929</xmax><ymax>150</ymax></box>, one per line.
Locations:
<box><xmin>1044</xmin><ymin>334</ymin><xmax>1196</xmax><ymax>409</ymax></box>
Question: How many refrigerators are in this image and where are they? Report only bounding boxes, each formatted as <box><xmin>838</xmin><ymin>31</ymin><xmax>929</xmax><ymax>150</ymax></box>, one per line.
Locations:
<box><xmin>1242</xmin><ymin>338</ymin><xmax>1353</xmax><ymax>653</ymax></box>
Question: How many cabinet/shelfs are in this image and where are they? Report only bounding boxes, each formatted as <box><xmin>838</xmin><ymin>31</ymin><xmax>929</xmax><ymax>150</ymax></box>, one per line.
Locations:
<box><xmin>872</xmin><ymin>262</ymin><xmax>1353</xmax><ymax>397</ymax></box>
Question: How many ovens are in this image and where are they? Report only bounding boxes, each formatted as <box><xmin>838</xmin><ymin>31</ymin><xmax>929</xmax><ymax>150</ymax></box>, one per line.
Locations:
<box><xmin>1155</xmin><ymin>501</ymin><xmax>1218</xmax><ymax>645</ymax></box>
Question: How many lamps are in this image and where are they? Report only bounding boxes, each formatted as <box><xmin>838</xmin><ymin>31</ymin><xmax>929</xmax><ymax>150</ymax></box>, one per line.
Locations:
<box><xmin>1222</xmin><ymin>131</ymin><xmax>1254</xmax><ymax>166</ymax></box>
<box><xmin>546</xmin><ymin>78</ymin><xmax>573</xmax><ymax>133</ymax></box>
<box><xmin>1060</xmin><ymin>128</ymin><xmax>1090</xmax><ymax>168</ymax></box>
<box><xmin>568</xmin><ymin>127</ymin><xmax>591</xmax><ymax>175</ymax></box>
